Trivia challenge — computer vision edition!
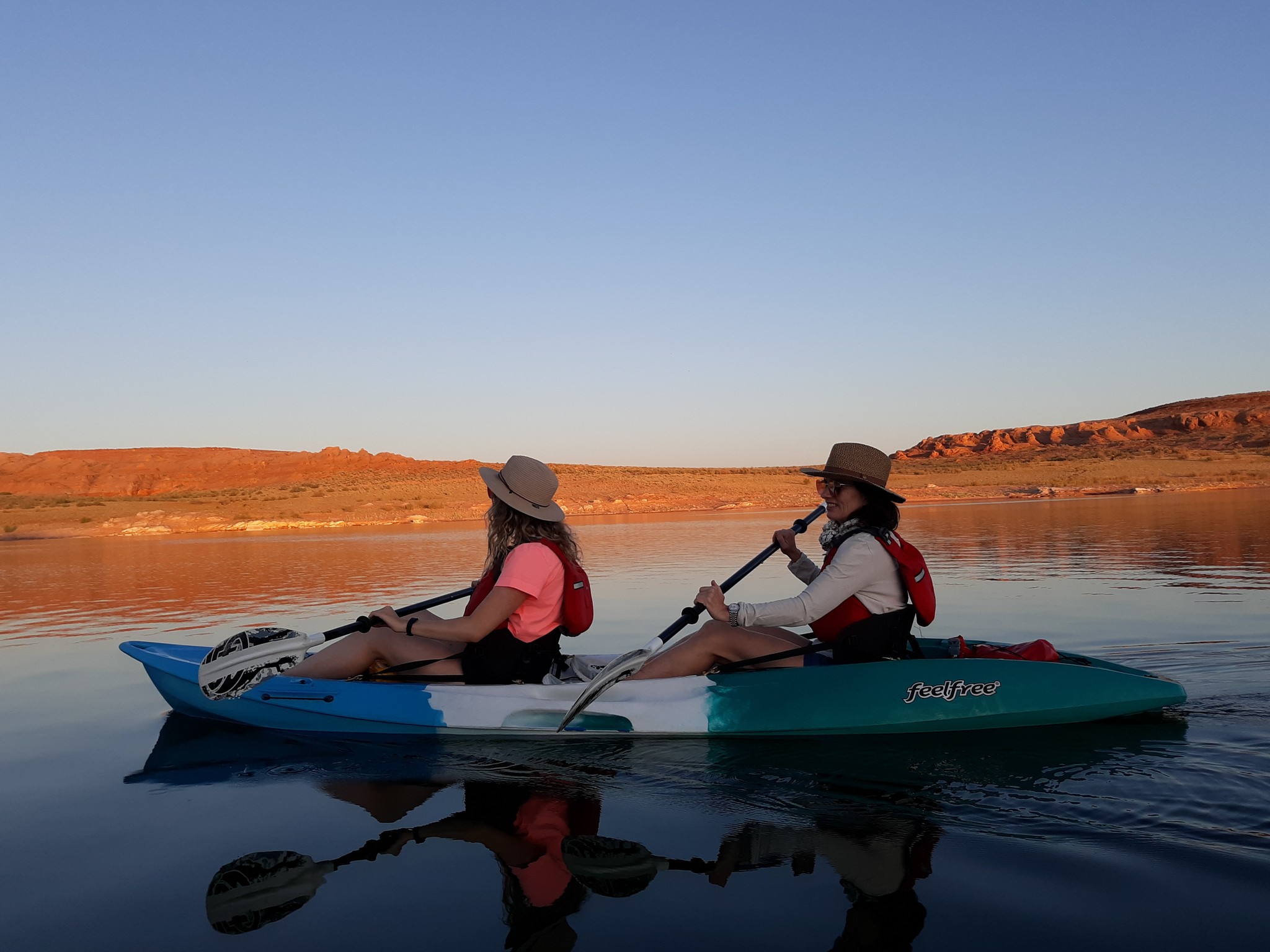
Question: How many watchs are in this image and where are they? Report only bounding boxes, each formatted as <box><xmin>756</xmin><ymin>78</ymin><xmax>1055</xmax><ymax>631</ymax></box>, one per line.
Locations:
<box><xmin>727</xmin><ymin>603</ymin><xmax>740</xmax><ymax>627</ymax></box>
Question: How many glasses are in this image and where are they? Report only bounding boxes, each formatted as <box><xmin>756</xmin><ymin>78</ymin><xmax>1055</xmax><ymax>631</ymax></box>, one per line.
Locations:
<box><xmin>816</xmin><ymin>479</ymin><xmax>855</xmax><ymax>497</ymax></box>
<box><xmin>487</xmin><ymin>486</ymin><xmax>498</xmax><ymax>499</ymax></box>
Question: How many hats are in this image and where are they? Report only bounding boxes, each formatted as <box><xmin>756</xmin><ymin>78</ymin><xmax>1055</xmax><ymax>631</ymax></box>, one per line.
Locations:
<box><xmin>479</xmin><ymin>455</ymin><xmax>565</xmax><ymax>521</ymax></box>
<box><xmin>800</xmin><ymin>442</ymin><xmax>906</xmax><ymax>504</ymax></box>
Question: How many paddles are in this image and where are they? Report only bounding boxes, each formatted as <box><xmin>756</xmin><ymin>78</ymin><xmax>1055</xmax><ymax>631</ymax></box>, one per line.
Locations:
<box><xmin>198</xmin><ymin>585</ymin><xmax>475</xmax><ymax>701</ymax></box>
<box><xmin>560</xmin><ymin>835</ymin><xmax>718</xmax><ymax>897</ymax></box>
<box><xmin>556</xmin><ymin>501</ymin><xmax>827</xmax><ymax>733</ymax></box>
<box><xmin>206</xmin><ymin>814</ymin><xmax>456</xmax><ymax>936</ymax></box>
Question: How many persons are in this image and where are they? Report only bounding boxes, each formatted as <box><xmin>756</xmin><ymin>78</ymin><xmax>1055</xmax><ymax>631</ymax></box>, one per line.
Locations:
<box><xmin>722</xmin><ymin>766</ymin><xmax>945</xmax><ymax>952</ymax></box>
<box><xmin>542</xmin><ymin>443</ymin><xmax>937</xmax><ymax>685</ymax></box>
<box><xmin>279</xmin><ymin>456</ymin><xmax>593</xmax><ymax>685</ymax></box>
<box><xmin>379</xmin><ymin>767</ymin><xmax>602</xmax><ymax>952</ymax></box>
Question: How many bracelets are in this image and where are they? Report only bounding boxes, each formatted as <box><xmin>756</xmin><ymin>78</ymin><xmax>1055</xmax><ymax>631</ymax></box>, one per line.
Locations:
<box><xmin>406</xmin><ymin>617</ymin><xmax>419</xmax><ymax>636</ymax></box>
<box><xmin>412</xmin><ymin>826</ymin><xmax>426</xmax><ymax>844</ymax></box>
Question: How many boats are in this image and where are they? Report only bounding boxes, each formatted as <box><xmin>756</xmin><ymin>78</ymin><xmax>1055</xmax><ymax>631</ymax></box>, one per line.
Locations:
<box><xmin>118</xmin><ymin>639</ymin><xmax>1192</xmax><ymax>739</ymax></box>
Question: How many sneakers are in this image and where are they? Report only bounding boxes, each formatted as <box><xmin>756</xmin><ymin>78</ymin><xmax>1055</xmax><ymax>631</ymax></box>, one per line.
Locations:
<box><xmin>571</xmin><ymin>656</ymin><xmax>607</xmax><ymax>682</ymax></box>
<box><xmin>542</xmin><ymin>674</ymin><xmax>570</xmax><ymax>685</ymax></box>
